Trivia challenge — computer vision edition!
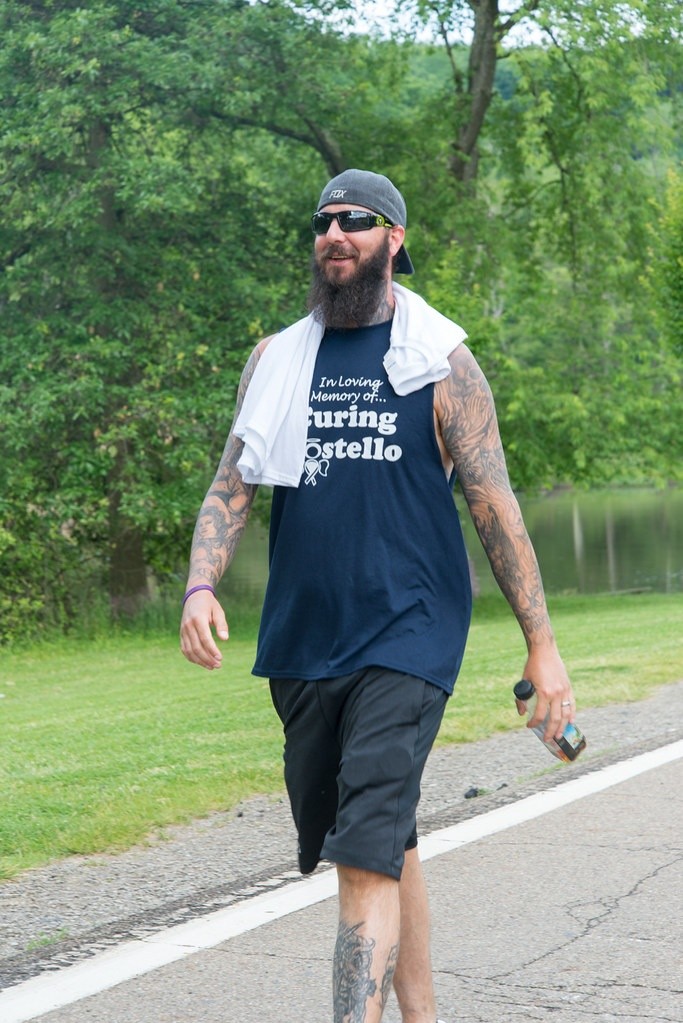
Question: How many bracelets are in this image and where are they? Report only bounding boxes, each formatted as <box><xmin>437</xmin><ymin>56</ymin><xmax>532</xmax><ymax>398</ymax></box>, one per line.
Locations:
<box><xmin>180</xmin><ymin>585</ymin><xmax>216</xmax><ymax>608</ymax></box>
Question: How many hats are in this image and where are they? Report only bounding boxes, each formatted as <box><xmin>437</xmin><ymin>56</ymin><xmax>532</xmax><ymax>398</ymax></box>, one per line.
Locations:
<box><xmin>317</xmin><ymin>169</ymin><xmax>414</xmax><ymax>274</ymax></box>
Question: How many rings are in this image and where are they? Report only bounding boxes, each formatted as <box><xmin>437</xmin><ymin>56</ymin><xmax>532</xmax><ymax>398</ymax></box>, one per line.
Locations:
<box><xmin>561</xmin><ymin>700</ymin><xmax>570</xmax><ymax>706</ymax></box>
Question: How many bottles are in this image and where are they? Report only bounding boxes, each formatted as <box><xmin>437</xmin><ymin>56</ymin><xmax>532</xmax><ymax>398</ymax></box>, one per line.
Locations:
<box><xmin>513</xmin><ymin>680</ymin><xmax>588</xmax><ymax>766</ymax></box>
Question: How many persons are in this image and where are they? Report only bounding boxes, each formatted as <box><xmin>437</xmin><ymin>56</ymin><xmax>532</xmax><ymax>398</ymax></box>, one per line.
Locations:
<box><xmin>178</xmin><ymin>168</ymin><xmax>577</xmax><ymax>1022</ymax></box>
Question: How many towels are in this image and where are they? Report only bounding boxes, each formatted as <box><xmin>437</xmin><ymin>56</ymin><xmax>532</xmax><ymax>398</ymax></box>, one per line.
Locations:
<box><xmin>232</xmin><ymin>280</ymin><xmax>469</xmax><ymax>489</ymax></box>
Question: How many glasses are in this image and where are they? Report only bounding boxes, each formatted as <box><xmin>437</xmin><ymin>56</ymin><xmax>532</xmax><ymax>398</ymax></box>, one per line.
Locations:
<box><xmin>311</xmin><ymin>210</ymin><xmax>393</xmax><ymax>234</ymax></box>
